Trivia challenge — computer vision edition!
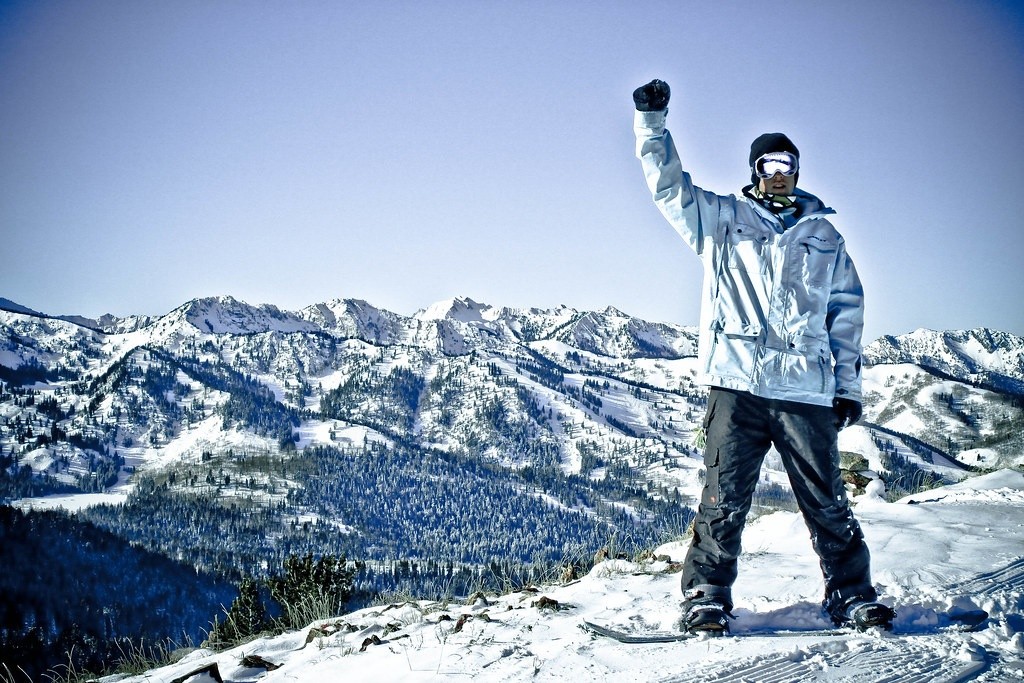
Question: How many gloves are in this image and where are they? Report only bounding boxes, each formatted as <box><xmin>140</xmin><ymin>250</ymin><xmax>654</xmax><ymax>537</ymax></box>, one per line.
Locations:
<box><xmin>633</xmin><ymin>79</ymin><xmax>670</xmax><ymax>112</ymax></box>
<box><xmin>832</xmin><ymin>397</ymin><xmax>862</xmax><ymax>432</ymax></box>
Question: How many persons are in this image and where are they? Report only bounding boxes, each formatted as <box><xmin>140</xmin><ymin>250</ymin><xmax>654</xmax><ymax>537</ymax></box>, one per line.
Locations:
<box><xmin>632</xmin><ymin>79</ymin><xmax>898</xmax><ymax>633</ymax></box>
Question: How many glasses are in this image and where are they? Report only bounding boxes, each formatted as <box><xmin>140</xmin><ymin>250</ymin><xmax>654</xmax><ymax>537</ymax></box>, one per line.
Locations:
<box><xmin>751</xmin><ymin>152</ymin><xmax>797</xmax><ymax>179</ymax></box>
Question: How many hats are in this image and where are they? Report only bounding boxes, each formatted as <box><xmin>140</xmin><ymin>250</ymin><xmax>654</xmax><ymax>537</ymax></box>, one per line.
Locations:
<box><xmin>749</xmin><ymin>133</ymin><xmax>799</xmax><ymax>190</ymax></box>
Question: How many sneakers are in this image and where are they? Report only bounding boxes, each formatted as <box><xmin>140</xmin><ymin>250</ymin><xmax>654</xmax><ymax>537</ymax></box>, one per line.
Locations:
<box><xmin>680</xmin><ymin>584</ymin><xmax>739</xmax><ymax>636</ymax></box>
<box><xmin>822</xmin><ymin>586</ymin><xmax>897</xmax><ymax>633</ymax></box>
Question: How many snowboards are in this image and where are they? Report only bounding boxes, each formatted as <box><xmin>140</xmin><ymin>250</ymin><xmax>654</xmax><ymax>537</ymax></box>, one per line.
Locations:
<box><xmin>583</xmin><ymin>607</ymin><xmax>989</xmax><ymax>644</ymax></box>
